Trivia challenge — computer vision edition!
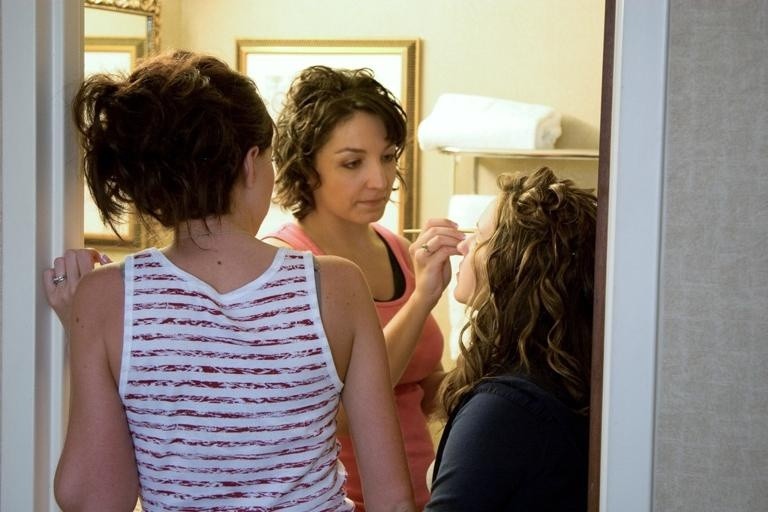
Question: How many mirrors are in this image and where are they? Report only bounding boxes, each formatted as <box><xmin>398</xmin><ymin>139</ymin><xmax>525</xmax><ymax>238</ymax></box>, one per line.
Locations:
<box><xmin>84</xmin><ymin>0</ymin><xmax>162</xmax><ymax>248</ymax></box>
<box><xmin>235</xmin><ymin>40</ymin><xmax>423</xmax><ymax>243</ymax></box>
<box><xmin>83</xmin><ymin>36</ymin><xmax>141</xmax><ymax>247</ymax></box>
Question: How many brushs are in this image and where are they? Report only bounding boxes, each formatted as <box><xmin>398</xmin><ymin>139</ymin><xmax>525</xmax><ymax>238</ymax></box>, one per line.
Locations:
<box><xmin>457</xmin><ymin>229</ymin><xmax>474</xmax><ymax>234</ymax></box>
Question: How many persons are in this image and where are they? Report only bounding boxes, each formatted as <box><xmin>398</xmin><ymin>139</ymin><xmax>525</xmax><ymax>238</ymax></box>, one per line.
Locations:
<box><xmin>420</xmin><ymin>164</ymin><xmax>597</xmax><ymax>512</ymax></box>
<box><xmin>40</xmin><ymin>50</ymin><xmax>414</xmax><ymax>511</ymax></box>
<box><xmin>261</xmin><ymin>63</ymin><xmax>468</xmax><ymax>511</ymax></box>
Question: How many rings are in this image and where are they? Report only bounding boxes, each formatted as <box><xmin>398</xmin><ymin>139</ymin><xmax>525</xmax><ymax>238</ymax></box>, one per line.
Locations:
<box><xmin>421</xmin><ymin>244</ymin><xmax>433</xmax><ymax>254</ymax></box>
<box><xmin>52</xmin><ymin>273</ymin><xmax>65</xmax><ymax>285</ymax></box>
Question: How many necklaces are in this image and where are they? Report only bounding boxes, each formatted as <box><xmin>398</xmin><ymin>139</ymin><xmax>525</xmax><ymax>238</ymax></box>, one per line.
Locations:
<box><xmin>172</xmin><ymin>229</ymin><xmax>252</xmax><ymax>241</ymax></box>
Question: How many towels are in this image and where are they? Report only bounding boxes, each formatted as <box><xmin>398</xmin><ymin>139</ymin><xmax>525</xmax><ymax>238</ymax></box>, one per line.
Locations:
<box><xmin>418</xmin><ymin>92</ymin><xmax>562</xmax><ymax>361</ymax></box>
